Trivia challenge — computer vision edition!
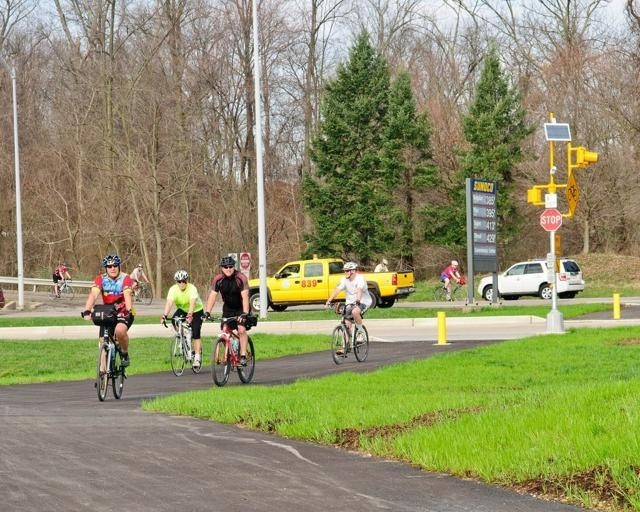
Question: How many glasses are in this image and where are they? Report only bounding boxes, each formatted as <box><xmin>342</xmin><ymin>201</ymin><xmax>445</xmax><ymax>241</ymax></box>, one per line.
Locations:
<box><xmin>107</xmin><ymin>265</ymin><xmax>118</xmax><ymax>268</ymax></box>
<box><xmin>223</xmin><ymin>266</ymin><xmax>234</xmax><ymax>269</ymax></box>
<box><xmin>177</xmin><ymin>281</ymin><xmax>185</xmax><ymax>284</ymax></box>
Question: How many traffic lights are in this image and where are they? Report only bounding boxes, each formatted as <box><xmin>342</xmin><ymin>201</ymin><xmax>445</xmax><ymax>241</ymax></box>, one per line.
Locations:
<box><xmin>578</xmin><ymin>147</ymin><xmax>600</xmax><ymax>166</ymax></box>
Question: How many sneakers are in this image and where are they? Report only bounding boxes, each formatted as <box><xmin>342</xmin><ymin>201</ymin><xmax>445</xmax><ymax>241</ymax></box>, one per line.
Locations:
<box><xmin>356</xmin><ymin>332</ymin><xmax>365</xmax><ymax>342</ymax></box>
<box><xmin>338</xmin><ymin>345</ymin><xmax>349</xmax><ymax>356</ymax></box>
<box><xmin>119</xmin><ymin>358</ymin><xmax>128</xmax><ymax>365</ymax></box>
<box><xmin>238</xmin><ymin>358</ymin><xmax>247</xmax><ymax>365</ymax></box>
<box><xmin>178</xmin><ymin>342</ymin><xmax>184</xmax><ymax>349</ymax></box>
<box><xmin>192</xmin><ymin>359</ymin><xmax>201</xmax><ymax>368</ymax></box>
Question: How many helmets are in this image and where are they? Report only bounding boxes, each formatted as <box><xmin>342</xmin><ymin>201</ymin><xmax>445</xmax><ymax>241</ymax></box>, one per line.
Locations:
<box><xmin>101</xmin><ymin>255</ymin><xmax>120</xmax><ymax>268</ymax></box>
<box><xmin>341</xmin><ymin>262</ymin><xmax>357</xmax><ymax>270</ymax></box>
<box><xmin>450</xmin><ymin>261</ymin><xmax>458</xmax><ymax>265</ymax></box>
<box><xmin>174</xmin><ymin>270</ymin><xmax>189</xmax><ymax>280</ymax></box>
<box><xmin>139</xmin><ymin>264</ymin><xmax>143</xmax><ymax>267</ymax></box>
<box><xmin>219</xmin><ymin>256</ymin><xmax>233</xmax><ymax>266</ymax></box>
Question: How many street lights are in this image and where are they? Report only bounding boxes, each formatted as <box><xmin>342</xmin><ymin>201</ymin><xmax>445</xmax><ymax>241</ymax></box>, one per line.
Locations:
<box><xmin>7</xmin><ymin>51</ymin><xmax>28</xmax><ymax>311</ymax></box>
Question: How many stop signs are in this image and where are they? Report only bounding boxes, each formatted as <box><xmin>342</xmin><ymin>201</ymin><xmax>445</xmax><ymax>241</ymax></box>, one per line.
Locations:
<box><xmin>239</xmin><ymin>253</ymin><xmax>252</xmax><ymax>270</ymax></box>
<box><xmin>539</xmin><ymin>209</ymin><xmax>562</xmax><ymax>232</ymax></box>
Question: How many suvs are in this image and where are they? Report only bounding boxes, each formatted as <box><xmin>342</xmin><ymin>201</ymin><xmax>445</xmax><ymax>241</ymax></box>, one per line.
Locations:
<box><xmin>476</xmin><ymin>259</ymin><xmax>585</xmax><ymax>304</ymax></box>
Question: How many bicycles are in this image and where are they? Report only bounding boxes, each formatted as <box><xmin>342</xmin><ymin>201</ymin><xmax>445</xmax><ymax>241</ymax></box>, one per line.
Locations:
<box><xmin>130</xmin><ymin>281</ymin><xmax>155</xmax><ymax>307</ymax></box>
<box><xmin>50</xmin><ymin>279</ymin><xmax>75</xmax><ymax>300</ymax></box>
<box><xmin>328</xmin><ymin>301</ymin><xmax>370</xmax><ymax>365</ymax></box>
<box><xmin>161</xmin><ymin>316</ymin><xmax>202</xmax><ymax>376</ymax></box>
<box><xmin>200</xmin><ymin>314</ymin><xmax>257</xmax><ymax>386</ymax></box>
<box><xmin>432</xmin><ymin>280</ymin><xmax>467</xmax><ymax>302</ymax></box>
<box><xmin>83</xmin><ymin>310</ymin><xmax>126</xmax><ymax>400</ymax></box>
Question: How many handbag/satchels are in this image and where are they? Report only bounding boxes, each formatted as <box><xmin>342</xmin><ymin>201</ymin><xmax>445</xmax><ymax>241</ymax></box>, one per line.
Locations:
<box><xmin>91</xmin><ymin>304</ymin><xmax>116</xmax><ymax>325</ymax></box>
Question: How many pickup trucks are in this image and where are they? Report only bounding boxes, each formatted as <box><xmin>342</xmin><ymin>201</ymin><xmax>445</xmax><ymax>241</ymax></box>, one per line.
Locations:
<box><xmin>245</xmin><ymin>256</ymin><xmax>415</xmax><ymax>308</ymax></box>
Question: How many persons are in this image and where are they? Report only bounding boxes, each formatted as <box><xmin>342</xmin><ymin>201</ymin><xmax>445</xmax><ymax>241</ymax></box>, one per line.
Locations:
<box><xmin>200</xmin><ymin>256</ymin><xmax>251</xmax><ymax>367</ymax></box>
<box><xmin>82</xmin><ymin>255</ymin><xmax>135</xmax><ymax>386</ymax></box>
<box><xmin>326</xmin><ymin>262</ymin><xmax>373</xmax><ymax>358</ymax></box>
<box><xmin>53</xmin><ymin>262</ymin><xmax>71</xmax><ymax>298</ymax></box>
<box><xmin>130</xmin><ymin>263</ymin><xmax>150</xmax><ymax>302</ymax></box>
<box><xmin>162</xmin><ymin>270</ymin><xmax>203</xmax><ymax>368</ymax></box>
<box><xmin>374</xmin><ymin>259</ymin><xmax>388</xmax><ymax>273</ymax></box>
<box><xmin>440</xmin><ymin>260</ymin><xmax>461</xmax><ymax>301</ymax></box>
<box><xmin>0</xmin><ymin>286</ymin><xmax>5</xmax><ymax>309</ymax></box>
